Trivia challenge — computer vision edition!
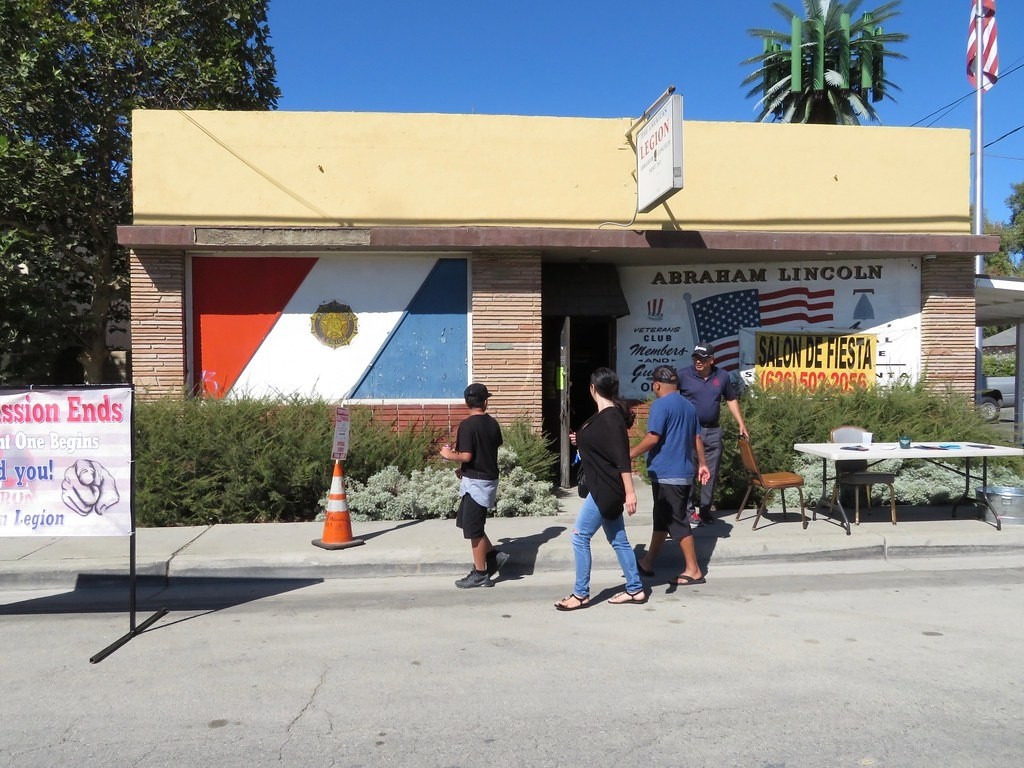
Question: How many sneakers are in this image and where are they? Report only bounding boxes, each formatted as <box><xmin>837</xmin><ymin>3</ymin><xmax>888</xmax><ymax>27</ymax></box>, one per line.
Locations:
<box><xmin>455</xmin><ymin>565</ymin><xmax>495</xmax><ymax>589</ymax></box>
<box><xmin>488</xmin><ymin>552</ymin><xmax>510</xmax><ymax>576</ymax></box>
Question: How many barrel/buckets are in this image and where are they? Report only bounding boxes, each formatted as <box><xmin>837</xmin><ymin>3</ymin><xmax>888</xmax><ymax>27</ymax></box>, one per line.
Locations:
<box><xmin>976</xmin><ymin>486</ymin><xmax>1024</xmax><ymax>524</ymax></box>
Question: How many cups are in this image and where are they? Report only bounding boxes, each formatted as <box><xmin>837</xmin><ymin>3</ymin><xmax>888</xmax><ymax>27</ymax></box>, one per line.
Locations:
<box><xmin>862</xmin><ymin>433</ymin><xmax>873</xmax><ymax>446</ymax></box>
<box><xmin>442</xmin><ymin>445</ymin><xmax>451</xmax><ymax>461</ymax></box>
<box><xmin>898</xmin><ymin>436</ymin><xmax>911</xmax><ymax>449</ymax></box>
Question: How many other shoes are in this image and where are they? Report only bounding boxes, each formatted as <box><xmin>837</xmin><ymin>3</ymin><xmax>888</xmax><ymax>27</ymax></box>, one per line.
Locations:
<box><xmin>699</xmin><ymin>511</ymin><xmax>714</xmax><ymax>525</ymax></box>
<box><xmin>688</xmin><ymin>512</ymin><xmax>703</xmax><ymax>526</ymax></box>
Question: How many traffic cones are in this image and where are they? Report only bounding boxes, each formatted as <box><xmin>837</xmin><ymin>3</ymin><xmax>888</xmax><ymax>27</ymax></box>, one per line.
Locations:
<box><xmin>309</xmin><ymin>460</ymin><xmax>365</xmax><ymax>550</ymax></box>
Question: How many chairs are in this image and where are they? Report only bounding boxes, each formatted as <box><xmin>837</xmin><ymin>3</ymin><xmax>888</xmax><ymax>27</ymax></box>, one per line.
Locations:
<box><xmin>828</xmin><ymin>425</ymin><xmax>896</xmax><ymax>526</ymax></box>
<box><xmin>734</xmin><ymin>434</ymin><xmax>807</xmax><ymax>532</ymax></box>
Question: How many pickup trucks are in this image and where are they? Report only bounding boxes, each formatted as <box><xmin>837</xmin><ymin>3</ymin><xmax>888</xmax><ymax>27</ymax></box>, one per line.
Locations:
<box><xmin>978</xmin><ymin>376</ymin><xmax>1016</xmax><ymax>423</ymax></box>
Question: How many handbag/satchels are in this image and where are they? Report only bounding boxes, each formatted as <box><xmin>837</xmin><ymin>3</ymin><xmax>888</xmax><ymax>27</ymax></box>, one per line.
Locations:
<box><xmin>577</xmin><ymin>464</ymin><xmax>589</xmax><ymax>498</ymax></box>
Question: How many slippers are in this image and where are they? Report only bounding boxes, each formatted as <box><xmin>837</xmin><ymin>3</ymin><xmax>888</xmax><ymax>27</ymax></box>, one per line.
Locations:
<box><xmin>669</xmin><ymin>575</ymin><xmax>706</xmax><ymax>585</ymax></box>
<box><xmin>637</xmin><ymin>562</ymin><xmax>655</xmax><ymax>576</ymax></box>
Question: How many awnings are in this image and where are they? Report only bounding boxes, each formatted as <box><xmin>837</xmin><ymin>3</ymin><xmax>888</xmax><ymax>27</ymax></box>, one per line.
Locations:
<box><xmin>541</xmin><ymin>261</ymin><xmax>629</xmax><ymax>319</ymax></box>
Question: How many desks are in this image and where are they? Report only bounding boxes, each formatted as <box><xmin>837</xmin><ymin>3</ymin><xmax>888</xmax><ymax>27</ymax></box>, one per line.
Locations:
<box><xmin>793</xmin><ymin>442</ymin><xmax>1024</xmax><ymax>536</ymax></box>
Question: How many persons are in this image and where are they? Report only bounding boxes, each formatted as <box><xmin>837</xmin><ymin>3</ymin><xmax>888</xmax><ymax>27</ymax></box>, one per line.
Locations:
<box><xmin>440</xmin><ymin>383</ymin><xmax>510</xmax><ymax>588</ymax></box>
<box><xmin>677</xmin><ymin>343</ymin><xmax>750</xmax><ymax>528</ymax></box>
<box><xmin>554</xmin><ymin>366</ymin><xmax>648</xmax><ymax>611</ymax></box>
<box><xmin>630</xmin><ymin>366</ymin><xmax>711</xmax><ymax>585</ymax></box>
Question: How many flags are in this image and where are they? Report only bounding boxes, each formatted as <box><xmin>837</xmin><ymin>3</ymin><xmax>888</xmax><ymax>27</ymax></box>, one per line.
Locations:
<box><xmin>966</xmin><ymin>0</ymin><xmax>999</xmax><ymax>92</ymax></box>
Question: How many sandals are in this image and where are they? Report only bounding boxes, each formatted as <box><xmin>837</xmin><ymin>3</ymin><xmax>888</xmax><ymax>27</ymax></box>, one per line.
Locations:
<box><xmin>554</xmin><ymin>594</ymin><xmax>590</xmax><ymax>611</ymax></box>
<box><xmin>608</xmin><ymin>590</ymin><xmax>646</xmax><ymax>603</ymax></box>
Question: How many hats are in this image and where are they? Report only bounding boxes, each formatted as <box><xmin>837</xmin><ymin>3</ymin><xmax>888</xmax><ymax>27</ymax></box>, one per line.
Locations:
<box><xmin>646</xmin><ymin>366</ymin><xmax>678</xmax><ymax>382</ymax></box>
<box><xmin>692</xmin><ymin>343</ymin><xmax>714</xmax><ymax>358</ymax></box>
<box><xmin>464</xmin><ymin>383</ymin><xmax>492</xmax><ymax>401</ymax></box>
